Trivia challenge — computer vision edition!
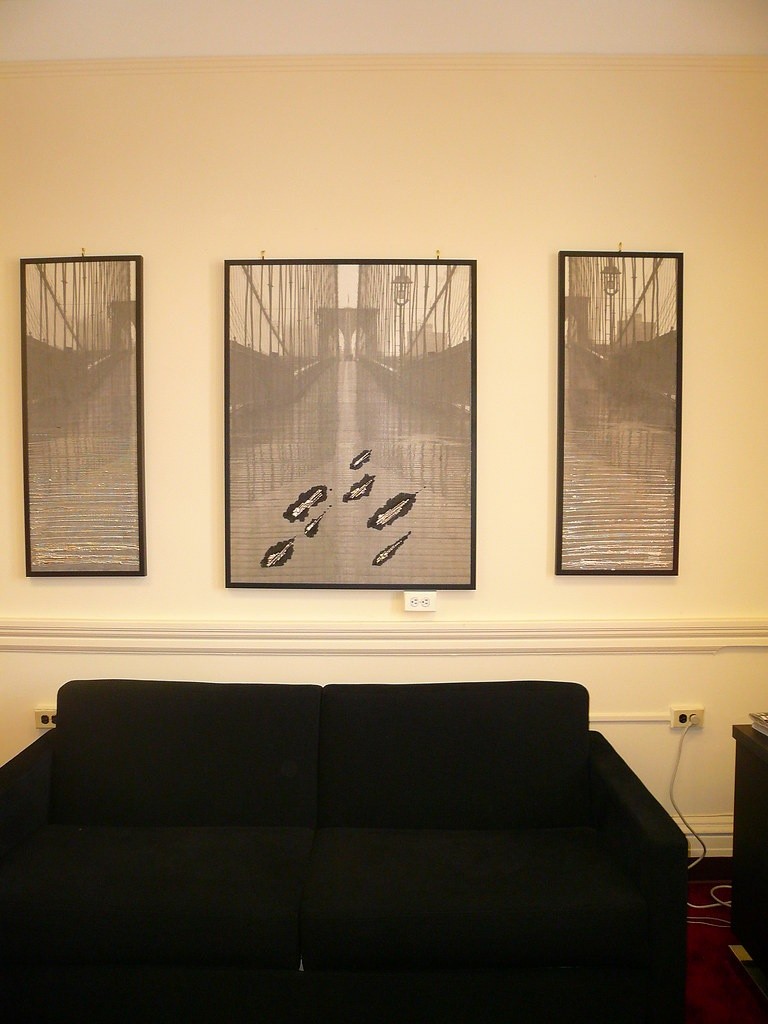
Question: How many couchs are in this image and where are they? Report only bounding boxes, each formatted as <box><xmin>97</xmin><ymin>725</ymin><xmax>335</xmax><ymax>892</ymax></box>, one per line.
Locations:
<box><xmin>0</xmin><ymin>678</ymin><xmax>688</xmax><ymax>1024</ymax></box>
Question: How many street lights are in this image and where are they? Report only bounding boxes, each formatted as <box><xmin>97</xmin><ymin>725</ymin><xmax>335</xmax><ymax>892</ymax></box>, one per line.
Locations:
<box><xmin>600</xmin><ymin>257</ymin><xmax>623</xmax><ymax>358</ymax></box>
<box><xmin>391</xmin><ymin>274</ymin><xmax>413</xmax><ymax>371</ymax></box>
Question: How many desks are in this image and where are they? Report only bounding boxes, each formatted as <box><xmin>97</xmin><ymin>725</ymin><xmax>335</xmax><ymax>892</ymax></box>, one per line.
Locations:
<box><xmin>729</xmin><ymin>722</ymin><xmax>768</xmax><ymax>1014</ymax></box>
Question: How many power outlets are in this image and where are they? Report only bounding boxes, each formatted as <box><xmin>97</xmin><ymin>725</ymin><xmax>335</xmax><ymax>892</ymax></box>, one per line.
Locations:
<box><xmin>405</xmin><ymin>591</ymin><xmax>436</xmax><ymax>612</ymax></box>
<box><xmin>33</xmin><ymin>709</ymin><xmax>58</xmax><ymax>729</ymax></box>
<box><xmin>671</xmin><ymin>706</ymin><xmax>706</xmax><ymax>728</ymax></box>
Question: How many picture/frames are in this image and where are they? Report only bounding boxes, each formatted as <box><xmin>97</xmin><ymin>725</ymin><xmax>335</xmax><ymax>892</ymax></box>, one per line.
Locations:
<box><xmin>555</xmin><ymin>250</ymin><xmax>684</xmax><ymax>576</ymax></box>
<box><xmin>225</xmin><ymin>258</ymin><xmax>478</xmax><ymax>592</ymax></box>
<box><xmin>20</xmin><ymin>254</ymin><xmax>148</xmax><ymax>578</ymax></box>
